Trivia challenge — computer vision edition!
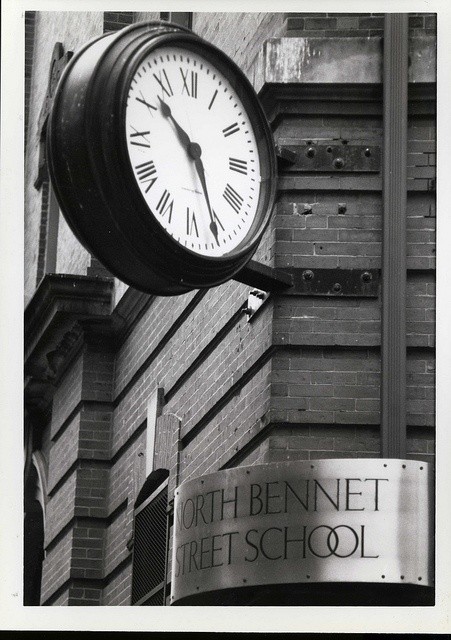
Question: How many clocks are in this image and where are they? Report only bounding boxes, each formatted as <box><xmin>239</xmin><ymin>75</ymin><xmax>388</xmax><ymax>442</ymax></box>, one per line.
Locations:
<box><xmin>47</xmin><ymin>20</ymin><xmax>278</xmax><ymax>297</ymax></box>
